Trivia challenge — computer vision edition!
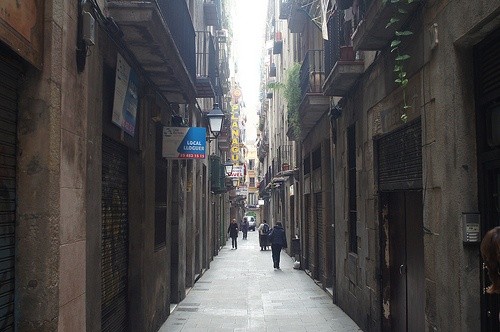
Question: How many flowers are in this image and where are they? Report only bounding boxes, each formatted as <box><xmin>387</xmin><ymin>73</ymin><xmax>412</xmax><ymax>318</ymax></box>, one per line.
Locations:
<box><xmin>343</xmin><ymin>34</ymin><xmax>352</xmax><ymax>47</ymax></box>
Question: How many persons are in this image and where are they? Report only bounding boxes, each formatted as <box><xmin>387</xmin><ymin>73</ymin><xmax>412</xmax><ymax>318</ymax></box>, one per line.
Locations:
<box><xmin>267</xmin><ymin>221</ymin><xmax>287</xmax><ymax>270</ymax></box>
<box><xmin>240</xmin><ymin>217</ymin><xmax>255</xmax><ymax>240</ymax></box>
<box><xmin>257</xmin><ymin>220</ymin><xmax>270</xmax><ymax>251</ymax></box>
<box><xmin>228</xmin><ymin>218</ymin><xmax>238</xmax><ymax>250</ymax></box>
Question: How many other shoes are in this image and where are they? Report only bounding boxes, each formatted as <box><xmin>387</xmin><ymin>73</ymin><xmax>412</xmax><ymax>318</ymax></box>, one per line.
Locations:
<box><xmin>273</xmin><ymin>266</ymin><xmax>280</xmax><ymax>269</ymax></box>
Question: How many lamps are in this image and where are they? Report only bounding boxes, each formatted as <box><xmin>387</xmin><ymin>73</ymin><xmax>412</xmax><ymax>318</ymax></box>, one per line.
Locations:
<box><xmin>205</xmin><ymin>102</ymin><xmax>225</xmax><ymax>141</ymax></box>
<box><xmin>224</xmin><ymin>158</ymin><xmax>233</xmax><ymax>176</ymax></box>
<box><xmin>231</xmin><ymin>177</ymin><xmax>239</xmax><ymax>187</ymax></box>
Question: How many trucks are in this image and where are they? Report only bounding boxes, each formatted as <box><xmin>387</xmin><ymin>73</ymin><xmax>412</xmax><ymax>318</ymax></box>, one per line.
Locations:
<box><xmin>241</xmin><ymin>216</ymin><xmax>256</xmax><ymax>231</ymax></box>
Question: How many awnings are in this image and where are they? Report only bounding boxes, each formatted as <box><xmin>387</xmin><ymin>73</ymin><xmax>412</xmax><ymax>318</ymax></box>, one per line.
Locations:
<box><xmin>265</xmin><ymin>182</ymin><xmax>281</xmax><ymax>190</ymax></box>
<box><xmin>272</xmin><ymin>178</ymin><xmax>287</xmax><ymax>182</ymax></box>
<box><xmin>275</xmin><ymin>170</ymin><xmax>295</xmax><ymax>177</ymax></box>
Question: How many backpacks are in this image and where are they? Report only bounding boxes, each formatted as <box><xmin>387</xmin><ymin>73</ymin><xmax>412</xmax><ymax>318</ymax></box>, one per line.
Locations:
<box><xmin>262</xmin><ymin>223</ymin><xmax>269</xmax><ymax>233</ymax></box>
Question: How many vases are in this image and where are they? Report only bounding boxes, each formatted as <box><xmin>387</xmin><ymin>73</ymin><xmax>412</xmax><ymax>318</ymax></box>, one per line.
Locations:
<box><xmin>340</xmin><ymin>47</ymin><xmax>354</xmax><ymax>61</ymax></box>
